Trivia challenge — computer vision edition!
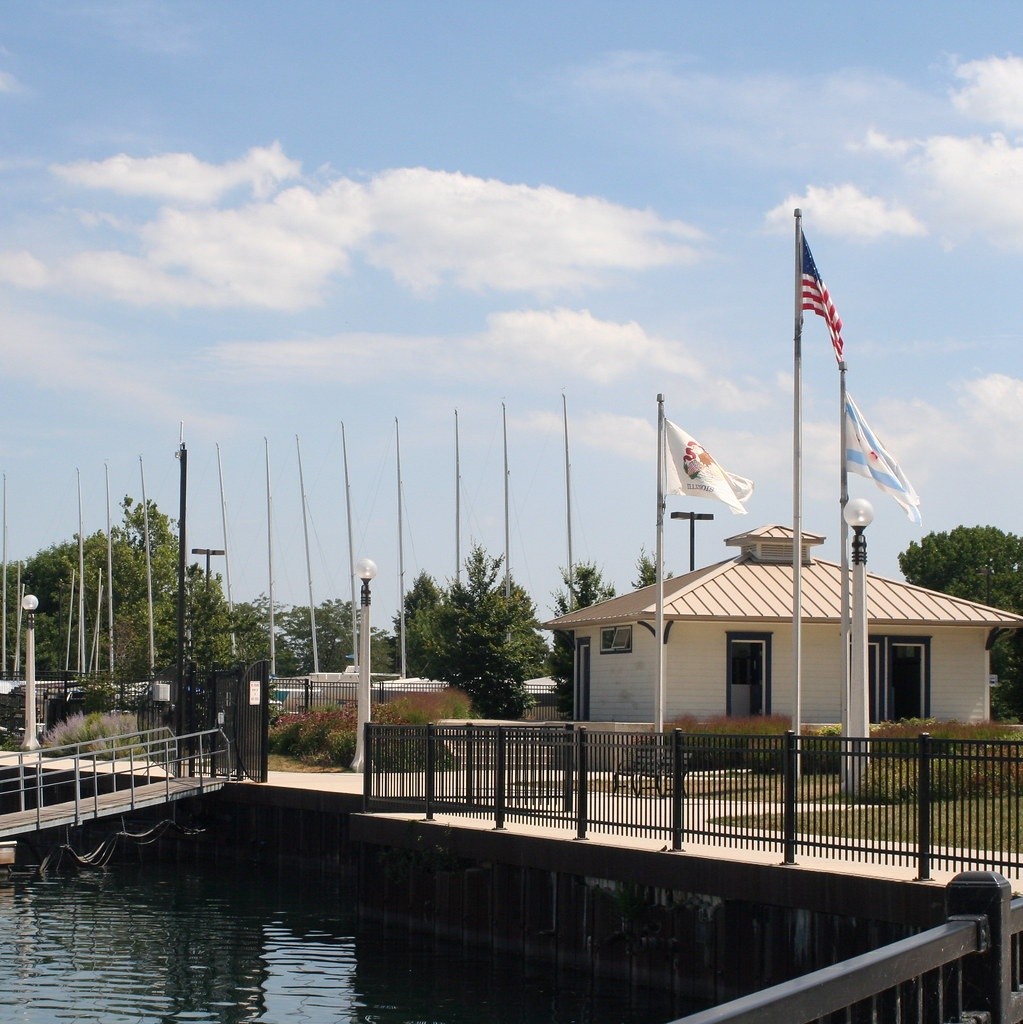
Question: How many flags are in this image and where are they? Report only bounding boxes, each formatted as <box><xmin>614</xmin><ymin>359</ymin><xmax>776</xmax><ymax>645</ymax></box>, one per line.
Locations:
<box><xmin>663</xmin><ymin>419</ymin><xmax>754</xmax><ymax>516</ymax></box>
<box><xmin>844</xmin><ymin>393</ymin><xmax>922</xmax><ymax>528</ymax></box>
<box><xmin>802</xmin><ymin>240</ymin><xmax>846</xmax><ymax>364</ymax></box>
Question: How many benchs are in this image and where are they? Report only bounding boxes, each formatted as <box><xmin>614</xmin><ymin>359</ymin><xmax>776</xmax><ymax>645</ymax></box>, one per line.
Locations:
<box><xmin>613</xmin><ymin>749</ymin><xmax>692</xmax><ymax>799</ymax></box>
